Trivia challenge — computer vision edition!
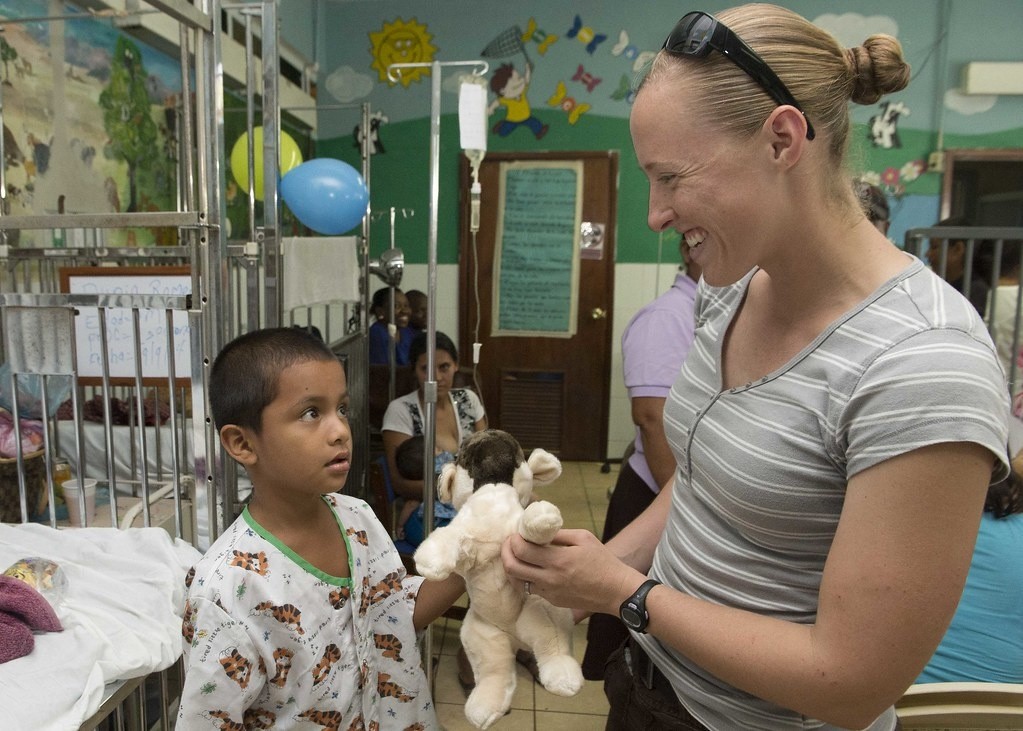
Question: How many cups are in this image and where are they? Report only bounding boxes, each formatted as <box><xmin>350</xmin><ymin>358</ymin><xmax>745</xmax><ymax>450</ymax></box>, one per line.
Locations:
<box><xmin>51</xmin><ymin>457</ymin><xmax>71</xmax><ymax>506</ymax></box>
<box><xmin>62</xmin><ymin>477</ymin><xmax>98</xmax><ymax>526</ymax></box>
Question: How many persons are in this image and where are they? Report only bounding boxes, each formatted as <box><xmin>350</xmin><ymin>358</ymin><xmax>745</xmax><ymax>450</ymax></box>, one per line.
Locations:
<box><xmin>169</xmin><ymin>322</ymin><xmax>498</xmax><ymax>730</ymax></box>
<box><xmin>394</xmin><ymin>289</ymin><xmax>442</xmax><ymax>357</ymax></box>
<box><xmin>361</xmin><ymin>286</ymin><xmax>416</xmax><ymax>366</ymax></box>
<box><xmin>375</xmin><ymin>330</ymin><xmax>500</xmax><ymax>575</ymax></box>
<box><xmin>392</xmin><ymin>436</ymin><xmax>475</xmax><ymax>512</ymax></box>
<box><xmin>500</xmin><ymin>0</ymin><xmax>1010</xmax><ymax>731</ymax></box>
<box><xmin>848</xmin><ymin>170</ymin><xmax>902</xmax><ymax>242</ymax></box>
<box><xmin>576</xmin><ymin>220</ymin><xmax>718</xmax><ymax>701</ymax></box>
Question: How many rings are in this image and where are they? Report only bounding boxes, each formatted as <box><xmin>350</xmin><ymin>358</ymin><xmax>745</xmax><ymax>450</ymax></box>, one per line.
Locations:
<box><xmin>523</xmin><ymin>581</ymin><xmax>532</xmax><ymax>595</ymax></box>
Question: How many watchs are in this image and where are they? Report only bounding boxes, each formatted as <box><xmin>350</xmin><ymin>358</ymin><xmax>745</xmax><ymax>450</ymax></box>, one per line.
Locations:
<box><xmin>615</xmin><ymin>577</ymin><xmax>661</xmax><ymax>634</ymax></box>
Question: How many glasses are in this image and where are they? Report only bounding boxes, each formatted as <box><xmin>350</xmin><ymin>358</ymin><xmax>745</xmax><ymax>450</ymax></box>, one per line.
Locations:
<box><xmin>661</xmin><ymin>11</ymin><xmax>816</xmax><ymax>142</ymax></box>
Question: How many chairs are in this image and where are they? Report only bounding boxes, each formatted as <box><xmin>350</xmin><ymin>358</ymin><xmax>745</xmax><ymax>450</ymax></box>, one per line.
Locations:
<box><xmin>378</xmin><ymin>455</ymin><xmax>418</xmax><ymax>559</ymax></box>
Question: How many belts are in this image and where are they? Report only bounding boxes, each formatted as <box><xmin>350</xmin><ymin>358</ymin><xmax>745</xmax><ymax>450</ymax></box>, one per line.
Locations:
<box><xmin>620</xmin><ymin>634</ymin><xmax>672</xmax><ymax>696</ymax></box>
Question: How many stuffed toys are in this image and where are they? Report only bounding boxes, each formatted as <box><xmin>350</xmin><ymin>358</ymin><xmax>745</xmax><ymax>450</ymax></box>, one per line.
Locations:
<box><xmin>408</xmin><ymin>426</ymin><xmax>586</xmax><ymax>730</ymax></box>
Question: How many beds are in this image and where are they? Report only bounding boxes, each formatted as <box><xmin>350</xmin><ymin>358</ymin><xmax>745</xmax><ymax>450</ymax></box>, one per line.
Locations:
<box><xmin>0</xmin><ymin>523</ymin><xmax>207</xmax><ymax>731</ymax></box>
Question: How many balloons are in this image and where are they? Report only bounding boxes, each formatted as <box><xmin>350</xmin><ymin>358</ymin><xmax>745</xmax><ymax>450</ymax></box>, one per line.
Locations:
<box><xmin>279</xmin><ymin>157</ymin><xmax>371</xmax><ymax>240</ymax></box>
<box><xmin>227</xmin><ymin>124</ymin><xmax>304</xmax><ymax>206</ymax></box>
